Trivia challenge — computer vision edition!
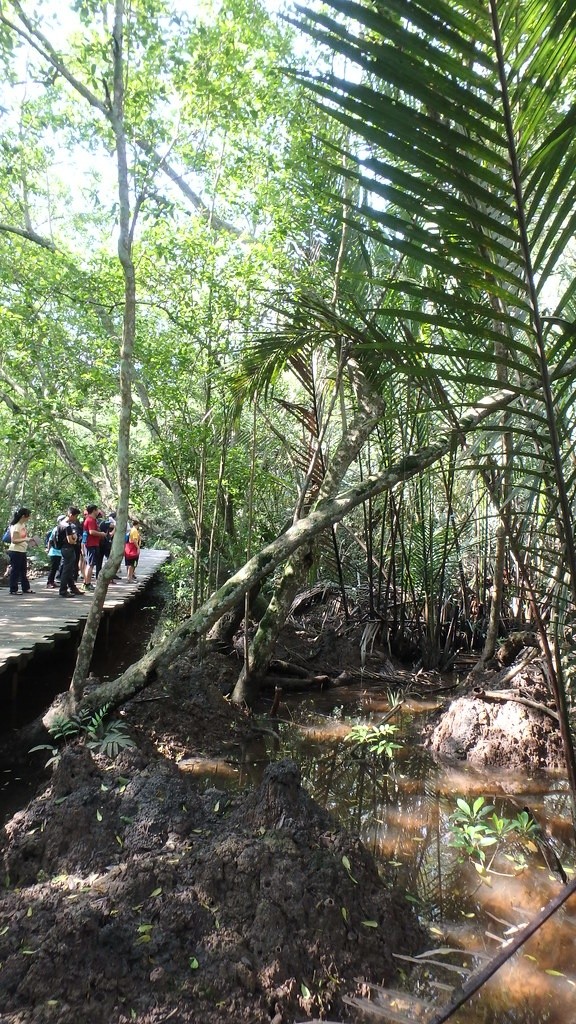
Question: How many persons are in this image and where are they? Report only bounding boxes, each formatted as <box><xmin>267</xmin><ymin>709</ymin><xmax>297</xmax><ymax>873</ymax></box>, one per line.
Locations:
<box><xmin>44</xmin><ymin>505</ymin><xmax>141</xmax><ymax>598</ymax></box>
<box><xmin>8</xmin><ymin>508</ymin><xmax>37</xmax><ymax>595</ymax></box>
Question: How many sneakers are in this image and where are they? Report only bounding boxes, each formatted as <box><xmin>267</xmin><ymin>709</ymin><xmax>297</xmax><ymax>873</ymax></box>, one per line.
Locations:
<box><xmin>70</xmin><ymin>589</ymin><xmax>85</xmax><ymax>595</ymax></box>
<box><xmin>82</xmin><ymin>583</ymin><xmax>96</xmax><ymax>592</ymax></box>
<box><xmin>59</xmin><ymin>591</ymin><xmax>75</xmax><ymax>598</ymax></box>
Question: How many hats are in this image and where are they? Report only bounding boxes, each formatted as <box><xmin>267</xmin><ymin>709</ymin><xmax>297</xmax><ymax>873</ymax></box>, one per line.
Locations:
<box><xmin>84</xmin><ymin>510</ymin><xmax>88</xmax><ymax>514</ymax></box>
<box><xmin>98</xmin><ymin>510</ymin><xmax>103</xmax><ymax>517</ymax></box>
<box><xmin>57</xmin><ymin>515</ymin><xmax>66</xmax><ymax>522</ymax></box>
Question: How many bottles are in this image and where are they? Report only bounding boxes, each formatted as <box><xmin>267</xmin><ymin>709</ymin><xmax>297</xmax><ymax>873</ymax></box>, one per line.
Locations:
<box><xmin>83</xmin><ymin>530</ymin><xmax>87</xmax><ymax>544</ymax></box>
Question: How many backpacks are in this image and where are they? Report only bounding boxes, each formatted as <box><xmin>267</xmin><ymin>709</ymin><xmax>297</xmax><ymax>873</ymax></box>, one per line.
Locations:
<box><xmin>44</xmin><ymin>530</ymin><xmax>53</xmax><ymax>548</ymax></box>
<box><xmin>49</xmin><ymin>525</ymin><xmax>67</xmax><ymax>550</ymax></box>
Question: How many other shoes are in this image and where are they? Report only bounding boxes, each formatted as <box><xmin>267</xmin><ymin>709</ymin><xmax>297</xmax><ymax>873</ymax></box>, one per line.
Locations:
<box><xmin>22</xmin><ymin>589</ymin><xmax>36</xmax><ymax>594</ymax></box>
<box><xmin>110</xmin><ymin>580</ymin><xmax>116</xmax><ymax>584</ymax></box>
<box><xmin>47</xmin><ymin>584</ymin><xmax>54</xmax><ymax>589</ymax></box>
<box><xmin>9</xmin><ymin>591</ymin><xmax>23</xmax><ymax>595</ymax></box>
<box><xmin>51</xmin><ymin>582</ymin><xmax>60</xmax><ymax>588</ymax></box>
<box><xmin>112</xmin><ymin>575</ymin><xmax>122</xmax><ymax>580</ymax></box>
<box><xmin>126</xmin><ymin>579</ymin><xmax>137</xmax><ymax>584</ymax></box>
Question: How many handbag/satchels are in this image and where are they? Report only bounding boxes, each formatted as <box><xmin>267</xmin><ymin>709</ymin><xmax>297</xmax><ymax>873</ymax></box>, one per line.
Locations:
<box><xmin>2</xmin><ymin>527</ymin><xmax>11</xmax><ymax>543</ymax></box>
<box><xmin>124</xmin><ymin>542</ymin><xmax>139</xmax><ymax>560</ymax></box>
